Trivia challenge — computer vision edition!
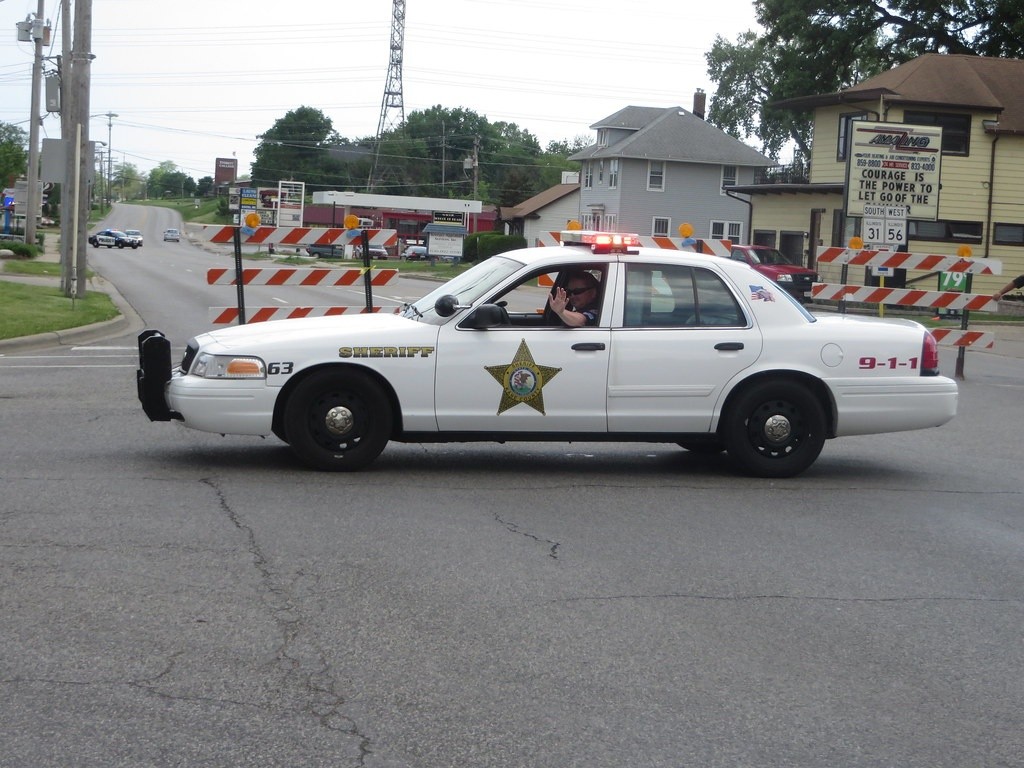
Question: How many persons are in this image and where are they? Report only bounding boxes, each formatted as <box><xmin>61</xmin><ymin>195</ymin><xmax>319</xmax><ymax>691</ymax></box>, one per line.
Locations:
<box><xmin>993</xmin><ymin>274</ymin><xmax>1024</xmax><ymax>301</ymax></box>
<box><xmin>548</xmin><ymin>272</ymin><xmax>602</xmax><ymax>328</ymax></box>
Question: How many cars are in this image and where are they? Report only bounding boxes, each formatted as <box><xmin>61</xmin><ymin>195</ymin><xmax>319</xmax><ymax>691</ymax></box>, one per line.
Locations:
<box><xmin>124</xmin><ymin>230</ymin><xmax>144</xmax><ymax>246</ymax></box>
<box><xmin>88</xmin><ymin>229</ymin><xmax>138</xmax><ymax>250</ymax></box>
<box><xmin>305</xmin><ymin>244</ymin><xmax>362</xmax><ymax>260</ymax></box>
<box><xmin>163</xmin><ymin>229</ymin><xmax>180</xmax><ymax>242</ymax></box>
<box><xmin>405</xmin><ymin>246</ymin><xmax>440</xmax><ymax>261</ymax></box>
<box><xmin>360</xmin><ymin>244</ymin><xmax>389</xmax><ymax>261</ymax></box>
<box><xmin>143</xmin><ymin>232</ymin><xmax>960</xmax><ymax>479</ymax></box>
<box><xmin>729</xmin><ymin>243</ymin><xmax>819</xmax><ymax>304</ymax></box>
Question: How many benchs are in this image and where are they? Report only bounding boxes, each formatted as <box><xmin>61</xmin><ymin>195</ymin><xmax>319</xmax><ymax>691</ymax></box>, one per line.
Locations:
<box><xmin>683</xmin><ymin>313</ymin><xmax>736</xmax><ymax>327</ymax></box>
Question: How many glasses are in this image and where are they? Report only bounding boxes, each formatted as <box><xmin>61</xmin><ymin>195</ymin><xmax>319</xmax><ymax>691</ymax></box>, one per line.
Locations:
<box><xmin>566</xmin><ymin>287</ymin><xmax>593</xmax><ymax>296</ymax></box>
